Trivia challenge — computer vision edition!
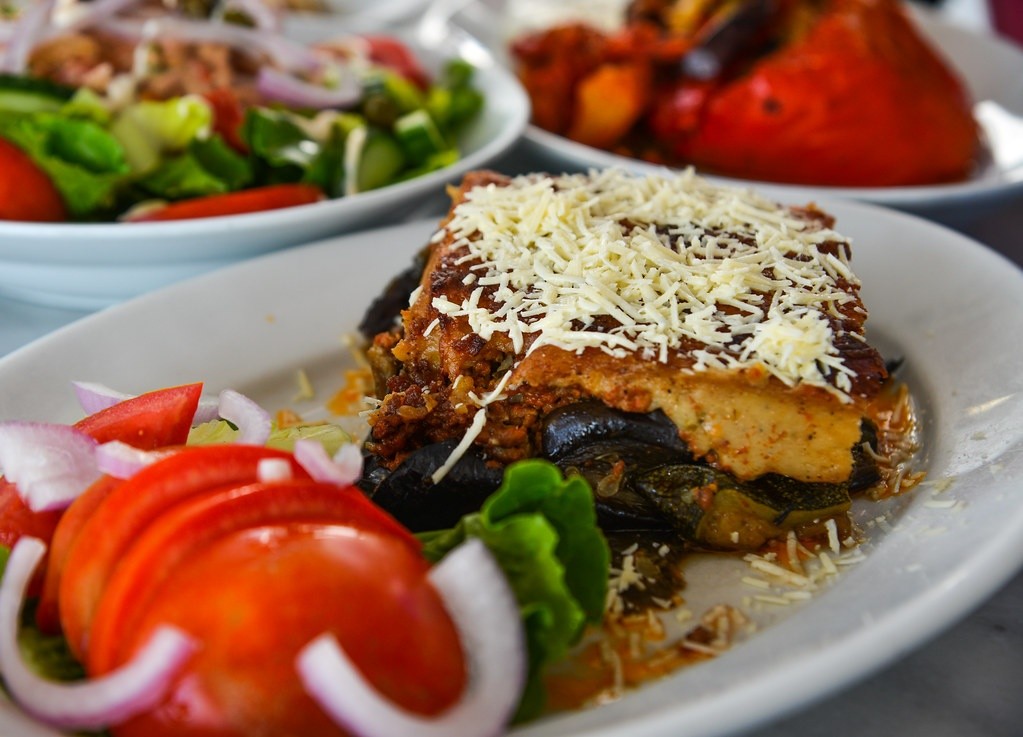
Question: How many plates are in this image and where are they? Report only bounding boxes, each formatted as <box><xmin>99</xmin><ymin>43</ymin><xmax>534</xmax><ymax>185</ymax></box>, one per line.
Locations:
<box><xmin>1</xmin><ymin>0</ymin><xmax>533</xmax><ymax>307</ymax></box>
<box><xmin>0</xmin><ymin>182</ymin><xmax>1019</xmax><ymax>733</ymax></box>
<box><xmin>436</xmin><ymin>2</ymin><xmax>1022</xmax><ymax>229</ymax></box>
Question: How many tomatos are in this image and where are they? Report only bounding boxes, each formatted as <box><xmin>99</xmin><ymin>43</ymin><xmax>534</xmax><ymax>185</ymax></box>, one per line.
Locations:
<box><xmin>0</xmin><ymin>374</ymin><xmax>466</xmax><ymax>737</ymax></box>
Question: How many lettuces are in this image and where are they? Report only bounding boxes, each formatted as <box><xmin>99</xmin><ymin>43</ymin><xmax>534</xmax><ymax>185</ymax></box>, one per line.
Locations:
<box><xmin>467</xmin><ymin>460</ymin><xmax>613</xmax><ymax>722</ymax></box>
